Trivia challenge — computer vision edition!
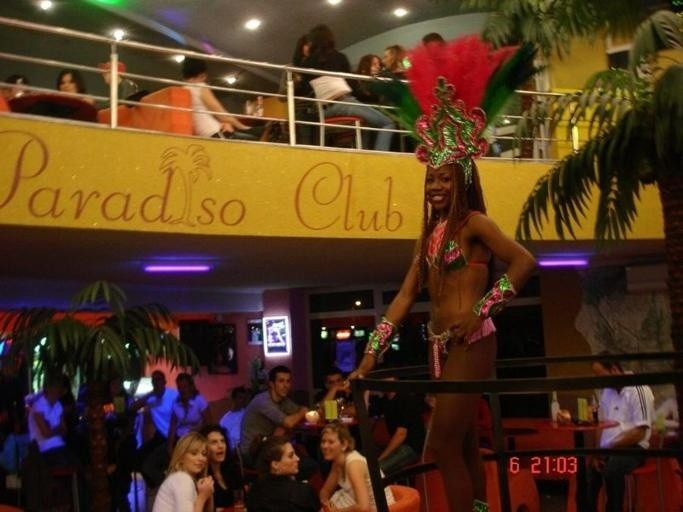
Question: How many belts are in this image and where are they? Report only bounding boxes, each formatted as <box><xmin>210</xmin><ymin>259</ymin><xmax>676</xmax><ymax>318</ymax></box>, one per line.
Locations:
<box><xmin>322</xmin><ymin>94</ymin><xmax>346</xmax><ymax>110</ymax></box>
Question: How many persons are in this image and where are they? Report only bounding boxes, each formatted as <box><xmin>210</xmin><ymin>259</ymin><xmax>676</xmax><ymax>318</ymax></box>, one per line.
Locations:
<box><xmin>289</xmin><ymin>24</ymin><xmax>447</xmax><ymax>150</ymax></box>
<box><xmin>347</xmin><ymin>146</ymin><xmax>537</xmax><ymax>511</ymax></box>
<box><xmin>26</xmin><ymin>364</ymin><xmax>427</xmax><ymax>511</ymax></box>
<box><xmin>100</xmin><ymin>60</ymin><xmax>138</xmax><ymax>105</ymax></box>
<box><xmin>580</xmin><ymin>350</ymin><xmax>654</xmax><ymax>511</ymax></box>
<box><xmin>181</xmin><ymin>55</ymin><xmax>255</xmax><ymax>139</ymax></box>
<box><xmin>57</xmin><ymin>67</ymin><xmax>97</xmax><ymax>106</ymax></box>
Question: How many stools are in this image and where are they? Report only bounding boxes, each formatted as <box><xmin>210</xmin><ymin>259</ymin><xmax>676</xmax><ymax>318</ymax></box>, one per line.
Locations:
<box><xmin>321</xmin><ymin>116</ymin><xmax>370</xmax><ymax>148</ymax></box>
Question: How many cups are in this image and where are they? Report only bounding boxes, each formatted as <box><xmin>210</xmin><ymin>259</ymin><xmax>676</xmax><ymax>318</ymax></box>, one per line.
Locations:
<box><xmin>654</xmin><ymin>412</ymin><xmax>664</xmax><ymax>432</ymax></box>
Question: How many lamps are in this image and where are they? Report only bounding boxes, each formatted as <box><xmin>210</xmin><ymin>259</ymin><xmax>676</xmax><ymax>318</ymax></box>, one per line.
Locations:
<box><xmin>533</xmin><ymin>254</ymin><xmax>592</xmax><ymax>268</ymax></box>
<box><xmin>142</xmin><ymin>261</ymin><xmax>214</xmax><ymax>275</ymax></box>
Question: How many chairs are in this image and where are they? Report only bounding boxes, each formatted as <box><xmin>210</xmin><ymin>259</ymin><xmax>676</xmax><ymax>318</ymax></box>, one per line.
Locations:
<box><xmin>95</xmin><ymin>86</ymin><xmax>195</xmax><ymax>137</ymax></box>
<box><xmin>211</xmin><ymin>402</ymin><xmax>682</xmax><ymax>512</ymax></box>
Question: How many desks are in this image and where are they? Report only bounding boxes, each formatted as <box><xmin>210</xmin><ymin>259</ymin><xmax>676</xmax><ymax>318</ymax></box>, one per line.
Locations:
<box><xmin>6</xmin><ymin>93</ymin><xmax>100</xmax><ymax>120</ymax></box>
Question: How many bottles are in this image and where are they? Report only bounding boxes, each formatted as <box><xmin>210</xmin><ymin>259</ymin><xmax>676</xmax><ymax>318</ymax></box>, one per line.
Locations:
<box><xmin>550</xmin><ymin>390</ymin><xmax>560</xmax><ymax>423</ymax></box>
<box><xmin>256</xmin><ymin>97</ymin><xmax>264</xmax><ymax>117</ymax></box>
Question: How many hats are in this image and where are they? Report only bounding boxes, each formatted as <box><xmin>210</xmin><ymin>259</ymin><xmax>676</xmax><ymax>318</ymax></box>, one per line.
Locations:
<box><xmin>416</xmin><ymin>77</ymin><xmax>489</xmax><ymax>190</ymax></box>
